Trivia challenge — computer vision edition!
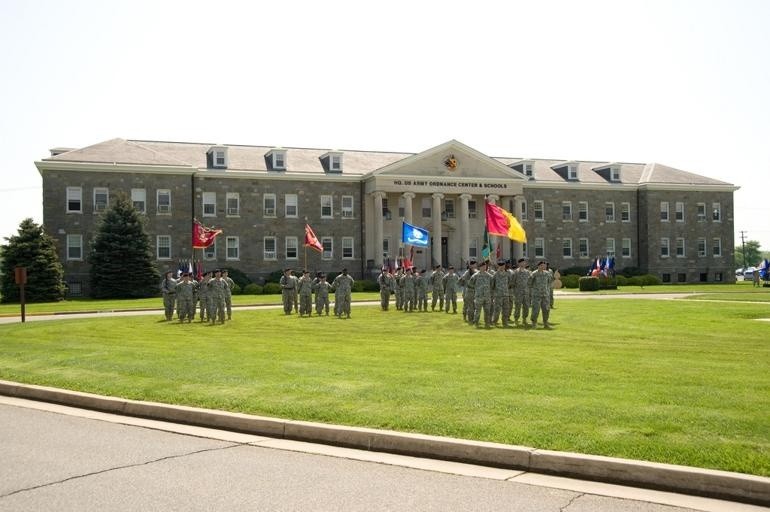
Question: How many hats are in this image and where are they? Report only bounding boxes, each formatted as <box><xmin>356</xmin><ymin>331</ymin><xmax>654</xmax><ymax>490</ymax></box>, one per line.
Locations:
<box><xmin>470</xmin><ymin>258</ymin><xmax>545</xmax><ymax>266</ymax></box>
<box><xmin>180</xmin><ymin>269</ymin><xmax>227</xmax><ymax>276</ymax></box>
<box><xmin>397</xmin><ymin>266</ymin><xmax>426</xmax><ymax>273</ymax></box>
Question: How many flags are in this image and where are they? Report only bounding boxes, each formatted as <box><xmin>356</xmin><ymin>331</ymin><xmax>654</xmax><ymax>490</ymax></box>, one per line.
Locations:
<box><xmin>191</xmin><ymin>220</ymin><xmax>223</xmax><ymax>249</ymax></box>
<box><xmin>304</xmin><ymin>223</ymin><xmax>324</xmax><ymax>253</ymax></box>
<box><xmin>586</xmin><ymin>252</ymin><xmax>616</xmax><ymax>276</ymax></box>
<box><xmin>402</xmin><ymin>221</ymin><xmax>430</xmax><ymax>249</ymax></box>
<box><xmin>176</xmin><ymin>258</ymin><xmax>203</xmax><ymax>283</ymax></box>
<box><xmin>484</xmin><ymin>198</ymin><xmax>528</xmax><ymax>244</ymax></box>
<box><xmin>481</xmin><ymin>222</ymin><xmax>500</xmax><ymax>265</ymax></box>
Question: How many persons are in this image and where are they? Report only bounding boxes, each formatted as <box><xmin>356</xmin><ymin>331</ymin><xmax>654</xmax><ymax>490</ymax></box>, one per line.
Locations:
<box><xmin>752</xmin><ymin>266</ymin><xmax>760</xmax><ymax>287</ymax></box>
<box><xmin>376</xmin><ymin>257</ymin><xmax>558</xmax><ymax>330</ymax></box>
<box><xmin>161</xmin><ymin>269</ymin><xmax>235</xmax><ymax>326</ymax></box>
<box><xmin>279</xmin><ymin>268</ymin><xmax>354</xmax><ymax>319</ymax></box>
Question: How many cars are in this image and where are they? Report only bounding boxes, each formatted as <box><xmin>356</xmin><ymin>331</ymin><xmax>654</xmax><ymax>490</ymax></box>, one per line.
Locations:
<box><xmin>748</xmin><ymin>266</ymin><xmax>755</xmax><ymax>271</ymax></box>
<box><xmin>736</xmin><ymin>268</ymin><xmax>744</xmax><ymax>276</ymax></box>
<box><xmin>744</xmin><ymin>270</ymin><xmax>753</xmax><ymax>280</ymax></box>
